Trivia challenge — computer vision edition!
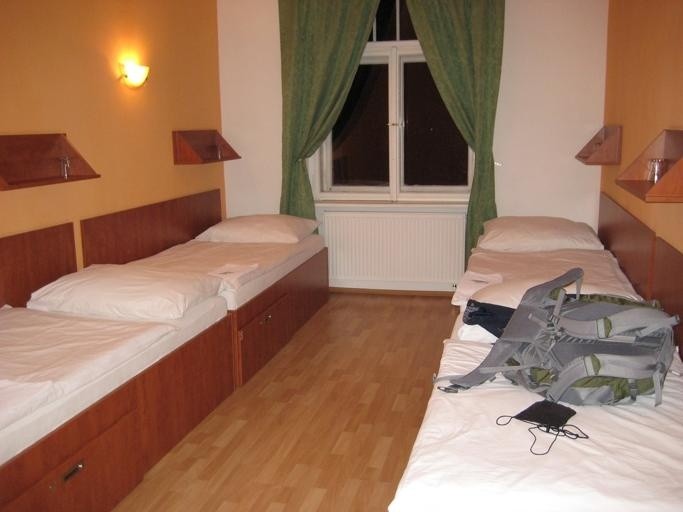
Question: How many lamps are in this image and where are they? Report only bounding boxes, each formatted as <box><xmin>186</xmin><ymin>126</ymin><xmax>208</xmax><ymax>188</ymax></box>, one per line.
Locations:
<box><xmin>116</xmin><ymin>55</ymin><xmax>150</xmax><ymax>89</ymax></box>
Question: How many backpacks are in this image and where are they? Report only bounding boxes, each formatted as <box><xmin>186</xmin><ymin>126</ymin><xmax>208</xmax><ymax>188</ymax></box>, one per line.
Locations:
<box><xmin>437</xmin><ymin>267</ymin><xmax>679</xmax><ymax>406</ymax></box>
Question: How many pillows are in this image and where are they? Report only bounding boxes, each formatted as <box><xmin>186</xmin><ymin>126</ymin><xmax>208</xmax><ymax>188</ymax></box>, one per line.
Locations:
<box><xmin>191</xmin><ymin>214</ymin><xmax>323</xmax><ymax>243</ymax></box>
<box><xmin>459</xmin><ymin>277</ymin><xmax>645</xmax><ymax>344</ymax></box>
<box><xmin>27</xmin><ymin>265</ymin><xmax>223</xmax><ymax>320</ymax></box>
<box><xmin>476</xmin><ymin>217</ymin><xmax>605</xmax><ymax>252</ymax></box>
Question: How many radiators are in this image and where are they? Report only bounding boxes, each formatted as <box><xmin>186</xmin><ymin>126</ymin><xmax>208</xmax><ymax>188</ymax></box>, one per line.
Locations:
<box><xmin>320</xmin><ymin>211</ymin><xmax>466</xmax><ymax>291</ymax></box>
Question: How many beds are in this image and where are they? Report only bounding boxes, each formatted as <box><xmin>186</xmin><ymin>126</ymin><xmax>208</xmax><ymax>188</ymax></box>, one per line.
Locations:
<box><xmin>0</xmin><ymin>291</ymin><xmax>237</xmax><ymax>512</ymax></box>
<box><xmin>451</xmin><ymin>249</ymin><xmax>645</xmax><ymax>307</ymax></box>
<box><xmin>387</xmin><ymin>275</ymin><xmax>683</xmax><ymax>512</ymax></box>
<box><xmin>121</xmin><ymin>213</ymin><xmax>329</xmax><ymax>392</ymax></box>
